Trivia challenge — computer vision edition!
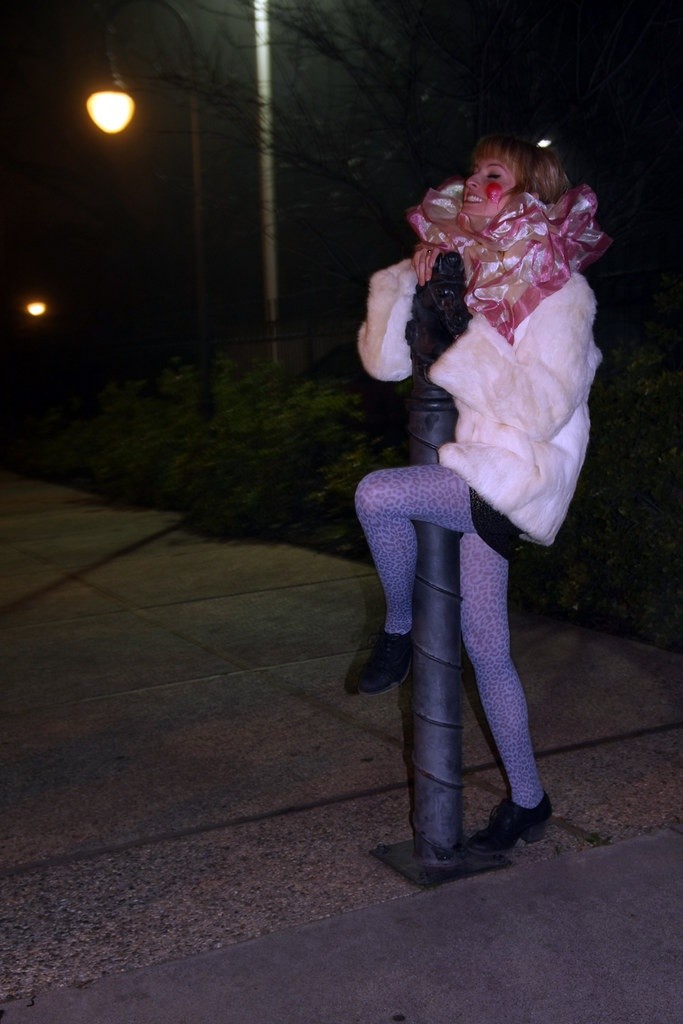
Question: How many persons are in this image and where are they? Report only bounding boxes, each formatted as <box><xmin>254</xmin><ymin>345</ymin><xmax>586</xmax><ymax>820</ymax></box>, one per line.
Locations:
<box><xmin>353</xmin><ymin>135</ymin><xmax>603</xmax><ymax>857</ymax></box>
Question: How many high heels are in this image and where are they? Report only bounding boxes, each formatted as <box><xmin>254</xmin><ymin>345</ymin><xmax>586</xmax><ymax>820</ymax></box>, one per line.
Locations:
<box><xmin>466</xmin><ymin>789</ymin><xmax>552</xmax><ymax>853</ymax></box>
<box><xmin>358</xmin><ymin>630</ymin><xmax>412</xmax><ymax>699</ymax></box>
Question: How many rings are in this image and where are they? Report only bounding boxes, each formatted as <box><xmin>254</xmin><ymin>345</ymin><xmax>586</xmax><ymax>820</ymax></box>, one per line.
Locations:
<box><xmin>427</xmin><ymin>249</ymin><xmax>433</xmax><ymax>254</ymax></box>
